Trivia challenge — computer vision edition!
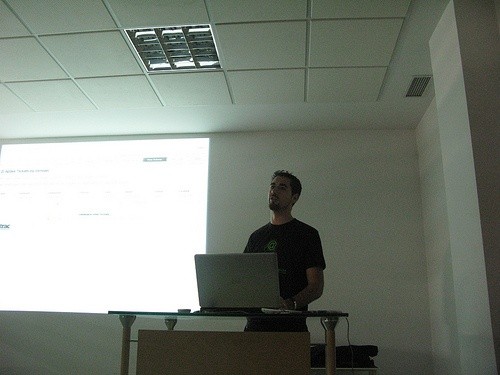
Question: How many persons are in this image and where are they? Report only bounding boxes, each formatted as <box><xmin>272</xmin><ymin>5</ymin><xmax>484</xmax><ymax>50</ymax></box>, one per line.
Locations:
<box><xmin>240</xmin><ymin>170</ymin><xmax>327</xmax><ymax>333</ymax></box>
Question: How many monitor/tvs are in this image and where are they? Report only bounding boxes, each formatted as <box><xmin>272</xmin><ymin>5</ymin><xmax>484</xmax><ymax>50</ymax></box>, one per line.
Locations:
<box><xmin>195</xmin><ymin>253</ymin><xmax>280</xmax><ymax>308</ymax></box>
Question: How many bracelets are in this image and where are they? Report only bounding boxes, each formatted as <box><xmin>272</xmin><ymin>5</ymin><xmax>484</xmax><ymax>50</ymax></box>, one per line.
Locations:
<box><xmin>291</xmin><ymin>297</ymin><xmax>298</xmax><ymax>311</ymax></box>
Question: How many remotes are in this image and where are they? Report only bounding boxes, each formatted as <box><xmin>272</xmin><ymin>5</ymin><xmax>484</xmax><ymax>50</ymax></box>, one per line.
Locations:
<box><xmin>261</xmin><ymin>308</ymin><xmax>302</xmax><ymax>314</ymax></box>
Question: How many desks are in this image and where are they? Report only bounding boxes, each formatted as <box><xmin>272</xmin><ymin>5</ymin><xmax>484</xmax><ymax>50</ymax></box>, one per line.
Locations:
<box><xmin>108</xmin><ymin>309</ymin><xmax>353</xmax><ymax>375</ymax></box>
